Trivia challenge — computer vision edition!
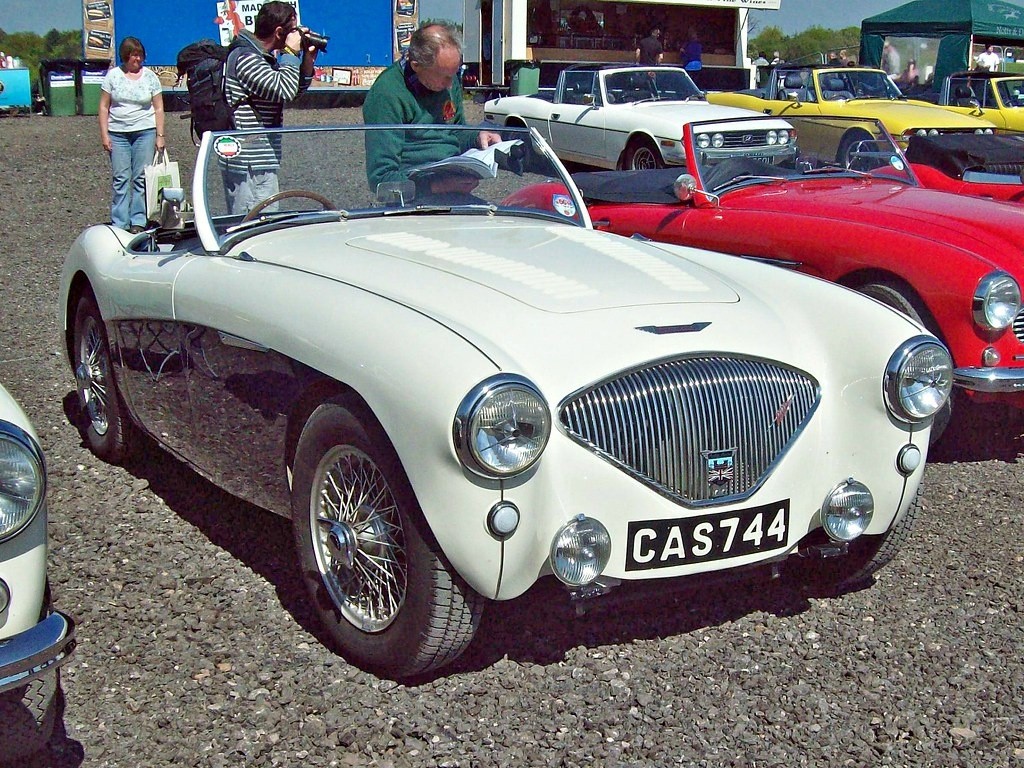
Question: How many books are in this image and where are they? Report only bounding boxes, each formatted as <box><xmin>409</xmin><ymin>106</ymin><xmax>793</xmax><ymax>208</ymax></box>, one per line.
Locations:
<box><xmin>421</xmin><ymin>140</ymin><xmax>525</xmax><ymax>176</ymax></box>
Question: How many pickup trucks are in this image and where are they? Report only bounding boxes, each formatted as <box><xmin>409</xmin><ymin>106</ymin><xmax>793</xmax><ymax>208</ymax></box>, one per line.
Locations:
<box><xmin>484</xmin><ymin>64</ymin><xmax>798</xmax><ymax>170</ymax></box>
<box><xmin>707</xmin><ymin>63</ymin><xmax>1024</xmax><ymax>167</ymax></box>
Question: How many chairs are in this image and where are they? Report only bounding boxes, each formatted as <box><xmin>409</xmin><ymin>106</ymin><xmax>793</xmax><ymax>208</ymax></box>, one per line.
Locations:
<box><xmin>777</xmin><ymin>74</ymin><xmax>815</xmax><ymax>104</ymax></box>
<box><xmin>954</xmin><ymin>86</ymin><xmax>981</xmax><ymax>108</ymax></box>
<box><xmin>986</xmin><ymin>86</ymin><xmax>1010</xmax><ymax>107</ymax></box>
<box><xmin>823</xmin><ymin>78</ymin><xmax>854</xmax><ymax>101</ymax></box>
<box><xmin>703</xmin><ymin>157</ymin><xmax>794</xmax><ymax>186</ymax></box>
<box><xmin>624</xmin><ymin>76</ymin><xmax>651</xmax><ymax>101</ymax></box>
<box><xmin>569</xmin><ymin>80</ymin><xmax>593</xmax><ymax>105</ymax></box>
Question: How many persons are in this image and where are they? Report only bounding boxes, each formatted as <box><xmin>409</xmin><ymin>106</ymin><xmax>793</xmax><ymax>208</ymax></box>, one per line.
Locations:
<box><xmin>634</xmin><ymin>30</ymin><xmax>705</xmax><ymax>90</ymax></box>
<box><xmin>99</xmin><ymin>37</ymin><xmax>165</xmax><ymax>233</ymax></box>
<box><xmin>364</xmin><ymin>24</ymin><xmax>504</xmax><ymax>207</ymax></box>
<box><xmin>752</xmin><ymin>40</ymin><xmax>1002</xmax><ymax>90</ymax></box>
<box><xmin>218</xmin><ymin>2</ymin><xmax>318</xmax><ymax>217</ymax></box>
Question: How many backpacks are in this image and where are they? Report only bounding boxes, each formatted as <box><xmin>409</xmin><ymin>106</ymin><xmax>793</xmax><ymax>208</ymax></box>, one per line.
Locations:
<box><xmin>176</xmin><ymin>40</ymin><xmax>257</xmax><ymax>147</ymax></box>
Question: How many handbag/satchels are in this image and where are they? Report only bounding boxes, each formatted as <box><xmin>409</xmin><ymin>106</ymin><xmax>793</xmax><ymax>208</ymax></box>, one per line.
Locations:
<box><xmin>143</xmin><ymin>149</ymin><xmax>184</xmax><ymax>220</ymax></box>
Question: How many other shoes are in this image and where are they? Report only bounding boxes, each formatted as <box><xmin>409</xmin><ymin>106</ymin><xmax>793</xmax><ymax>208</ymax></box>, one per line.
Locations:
<box><xmin>129</xmin><ymin>225</ymin><xmax>144</xmax><ymax>233</ymax></box>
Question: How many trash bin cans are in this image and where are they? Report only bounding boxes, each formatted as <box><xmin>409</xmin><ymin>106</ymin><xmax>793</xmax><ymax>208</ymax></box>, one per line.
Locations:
<box><xmin>41</xmin><ymin>59</ymin><xmax>78</xmax><ymax>115</ymax></box>
<box><xmin>508</xmin><ymin>59</ymin><xmax>540</xmax><ymax>96</ymax></box>
<box><xmin>76</xmin><ymin>59</ymin><xmax>111</xmax><ymax>115</ymax></box>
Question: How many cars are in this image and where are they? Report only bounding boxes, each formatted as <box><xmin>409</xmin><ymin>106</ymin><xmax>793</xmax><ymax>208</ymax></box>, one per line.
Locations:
<box><xmin>61</xmin><ymin>122</ymin><xmax>960</xmax><ymax>684</ymax></box>
<box><xmin>0</xmin><ymin>379</ymin><xmax>75</xmax><ymax>767</ymax></box>
<box><xmin>491</xmin><ymin>111</ymin><xmax>1024</xmax><ymax>440</ymax></box>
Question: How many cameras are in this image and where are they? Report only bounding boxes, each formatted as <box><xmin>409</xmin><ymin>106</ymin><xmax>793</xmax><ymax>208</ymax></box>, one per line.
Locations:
<box><xmin>295</xmin><ymin>28</ymin><xmax>330</xmax><ymax>54</ymax></box>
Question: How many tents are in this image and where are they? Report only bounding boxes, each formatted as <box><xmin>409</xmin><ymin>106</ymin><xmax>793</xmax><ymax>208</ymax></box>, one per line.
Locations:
<box><xmin>858</xmin><ymin>0</ymin><xmax>1024</xmax><ymax>103</ymax></box>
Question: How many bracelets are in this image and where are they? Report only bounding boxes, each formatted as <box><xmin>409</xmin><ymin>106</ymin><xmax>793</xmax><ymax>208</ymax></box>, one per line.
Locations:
<box><xmin>157</xmin><ymin>134</ymin><xmax>166</xmax><ymax>137</ymax></box>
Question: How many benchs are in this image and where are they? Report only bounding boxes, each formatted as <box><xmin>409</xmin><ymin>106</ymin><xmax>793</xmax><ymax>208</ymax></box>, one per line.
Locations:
<box><xmin>170</xmin><ymin>191</ymin><xmax>489</xmax><ymax>251</ymax></box>
